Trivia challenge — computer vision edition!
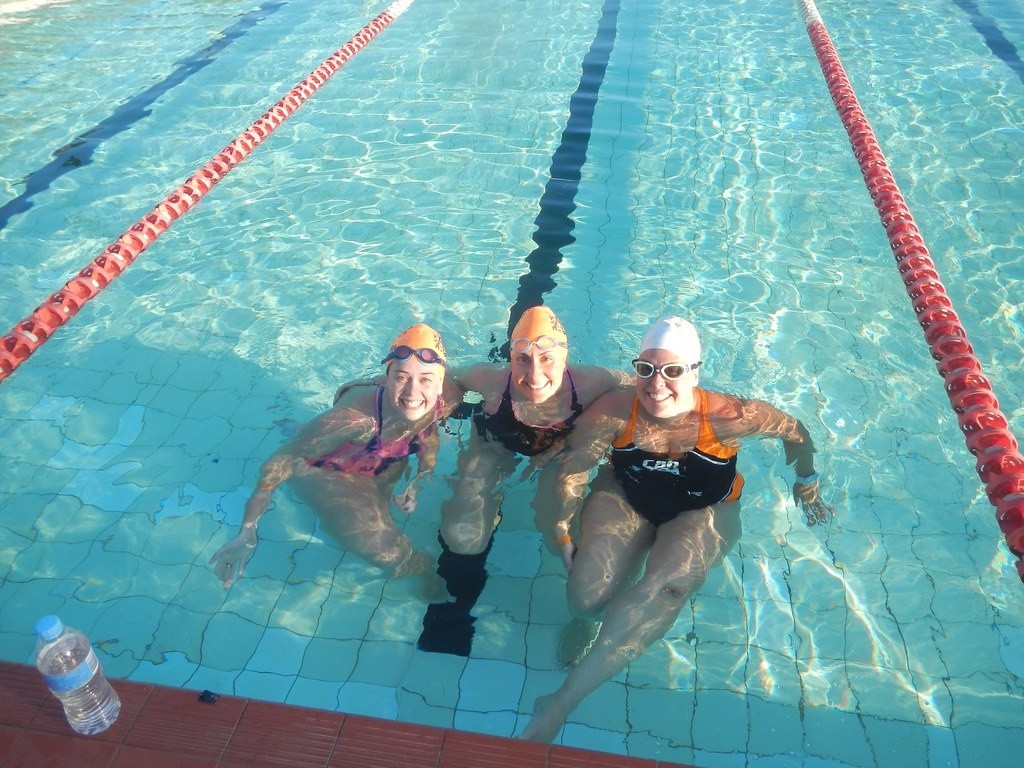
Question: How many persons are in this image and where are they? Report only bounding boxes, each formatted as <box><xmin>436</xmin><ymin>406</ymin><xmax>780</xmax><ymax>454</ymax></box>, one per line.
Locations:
<box><xmin>205</xmin><ymin>323</ymin><xmax>450</xmax><ymax>589</ymax></box>
<box><xmin>331</xmin><ymin>306</ymin><xmax>642</xmax><ymax>558</ymax></box>
<box><xmin>518</xmin><ymin>316</ymin><xmax>835</xmax><ymax>744</ymax></box>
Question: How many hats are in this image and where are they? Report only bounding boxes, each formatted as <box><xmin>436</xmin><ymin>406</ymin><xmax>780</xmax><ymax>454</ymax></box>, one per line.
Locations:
<box><xmin>511</xmin><ymin>306</ymin><xmax>568</xmax><ymax>358</ymax></box>
<box><xmin>387</xmin><ymin>323</ymin><xmax>447</xmax><ymax>367</ymax></box>
<box><xmin>639</xmin><ymin>316</ymin><xmax>701</xmax><ymax>374</ymax></box>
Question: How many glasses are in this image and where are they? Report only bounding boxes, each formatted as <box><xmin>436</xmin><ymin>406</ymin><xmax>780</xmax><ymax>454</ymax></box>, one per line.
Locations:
<box><xmin>632</xmin><ymin>359</ymin><xmax>702</xmax><ymax>380</ymax></box>
<box><xmin>510</xmin><ymin>335</ymin><xmax>568</xmax><ymax>353</ymax></box>
<box><xmin>381</xmin><ymin>345</ymin><xmax>447</xmax><ymax>368</ymax></box>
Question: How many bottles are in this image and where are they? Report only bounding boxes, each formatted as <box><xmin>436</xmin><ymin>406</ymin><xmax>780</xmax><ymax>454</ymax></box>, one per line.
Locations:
<box><xmin>34</xmin><ymin>615</ymin><xmax>121</xmax><ymax>736</ymax></box>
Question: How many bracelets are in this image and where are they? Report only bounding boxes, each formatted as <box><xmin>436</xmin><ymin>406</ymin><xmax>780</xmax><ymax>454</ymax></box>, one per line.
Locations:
<box><xmin>795</xmin><ymin>470</ymin><xmax>819</xmax><ymax>485</ymax></box>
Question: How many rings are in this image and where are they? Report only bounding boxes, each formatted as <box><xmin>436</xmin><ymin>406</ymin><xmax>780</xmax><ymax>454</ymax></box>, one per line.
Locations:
<box><xmin>225</xmin><ymin>564</ymin><xmax>232</xmax><ymax>569</ymax></box>
